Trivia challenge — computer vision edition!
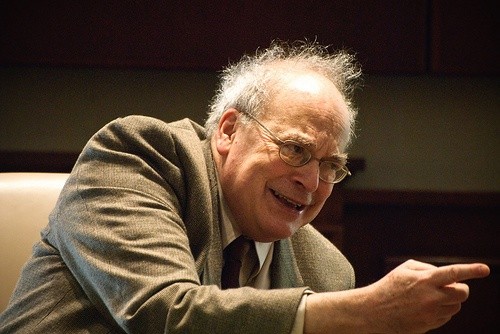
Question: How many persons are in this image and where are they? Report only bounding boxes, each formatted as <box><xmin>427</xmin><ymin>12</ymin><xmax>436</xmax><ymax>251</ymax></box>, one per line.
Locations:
<box><xmin>0</xmin><ymin>42</ymin><xmax>490</xmax><ymax>334</ymax></box>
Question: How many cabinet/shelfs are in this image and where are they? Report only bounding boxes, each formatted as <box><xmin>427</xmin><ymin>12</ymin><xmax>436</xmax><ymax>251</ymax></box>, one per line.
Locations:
<box><xmin>0</xmin><ymin>1</ymin><xmax>499</xmax><ymax>74</ymax></box>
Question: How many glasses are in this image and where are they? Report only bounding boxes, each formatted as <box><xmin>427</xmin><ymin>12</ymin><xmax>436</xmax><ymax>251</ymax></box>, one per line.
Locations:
<box><xmin>238</xmin><ymin>108</ymin><xmax>352</xmax><ymax>184</ymax></box>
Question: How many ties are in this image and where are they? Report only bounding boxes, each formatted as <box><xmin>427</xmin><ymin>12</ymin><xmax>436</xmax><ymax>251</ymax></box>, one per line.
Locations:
<box><xmin>221</xmin><ymin>242</ymin><xmax>249</xmax><ymax>288</ymax></box>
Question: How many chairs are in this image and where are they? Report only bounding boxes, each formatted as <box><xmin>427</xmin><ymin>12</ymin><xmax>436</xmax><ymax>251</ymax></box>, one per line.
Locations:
<box><xmin>0</xmin><ymin>173</ymin><xmax>71</xmax><ymax>314</ymax></box>
